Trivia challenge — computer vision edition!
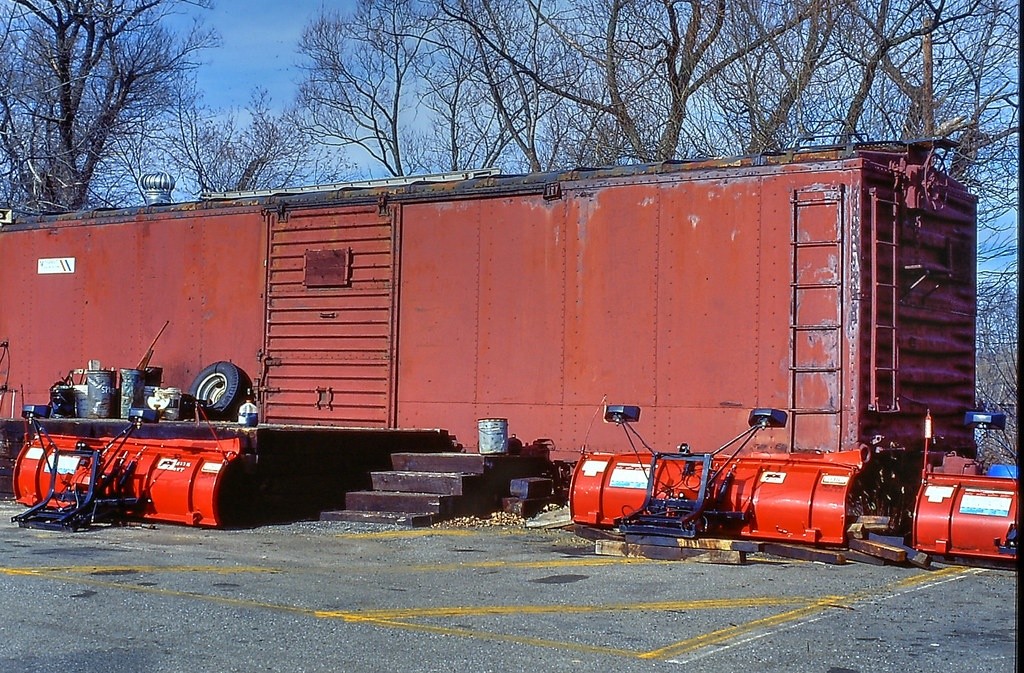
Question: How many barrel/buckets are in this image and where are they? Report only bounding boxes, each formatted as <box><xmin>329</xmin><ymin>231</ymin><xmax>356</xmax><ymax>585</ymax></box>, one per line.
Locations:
<box><xmin>144</xmin><ymin>367</ymin><xmax>164</xmax><ymax>387</ymax></box>
<box><xmin>49</xmin><ymin>391</ymin><xmax>75</xmax><ymax>418</ymax></box>
<box><xmin>74</xmin><ymin>384</ymin><xmax>89</xmax><ymax>418</ymax></box>
<box><xmin>119</xmin><ymin>367</ymin><xmax>145</xmax><ymax>418</ymax></box>
<box><xmin>87</xmin><ymin>370</ymin><xmax>117</xmax><ymax>419</ymax></box>
<box><xmin>478</xmin><ymin>418</ymin><xmax>509</xmax><ymax>455</ymax></box>
<box><xmin>144</xmin><ymin>386</ymin><xmax>155</xmax><ymax>408</ymax></box>
<box><xmin>165</xmin><ymin>388</ymin><xmax>182</xmax><ymax>420</ymax></box>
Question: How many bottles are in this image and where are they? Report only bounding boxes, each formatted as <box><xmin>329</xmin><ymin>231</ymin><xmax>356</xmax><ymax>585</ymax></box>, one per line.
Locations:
<box><xmin>508</xmin><ymin>434</ymin><xmax>523</xmax><ymax>456</ymax></box>
<box><xmin>238</xmin><ymin>400</ymin><xmax>258</xmax><ymax>427</ymax></box>
